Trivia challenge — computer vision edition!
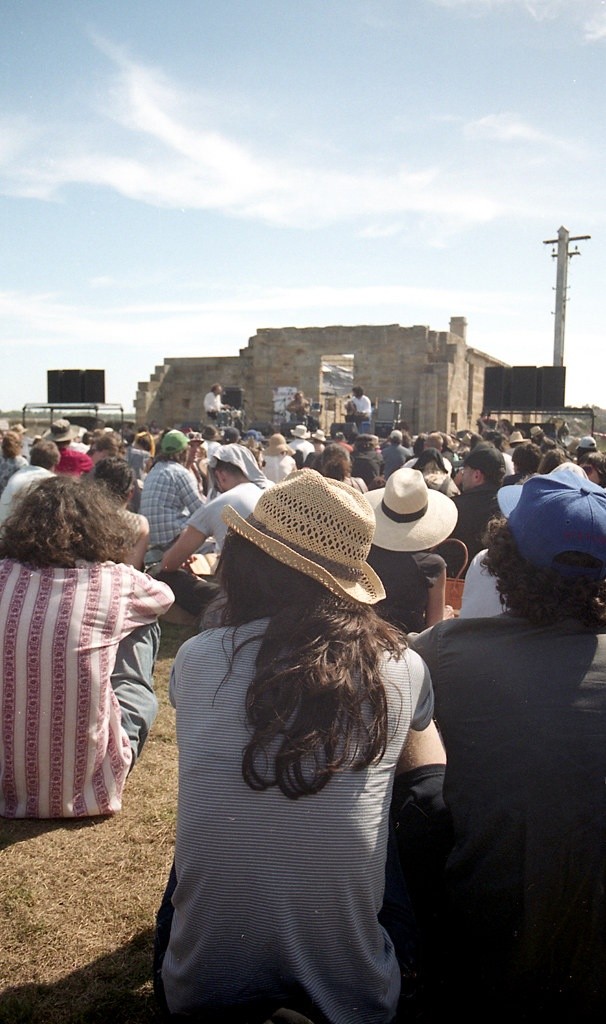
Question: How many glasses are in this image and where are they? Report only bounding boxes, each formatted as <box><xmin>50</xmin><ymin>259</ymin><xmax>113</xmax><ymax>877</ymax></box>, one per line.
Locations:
<box><xmin>583</xmin><ymin>466</ymin><xmax>593</xmax><ymax>476</ymax></box>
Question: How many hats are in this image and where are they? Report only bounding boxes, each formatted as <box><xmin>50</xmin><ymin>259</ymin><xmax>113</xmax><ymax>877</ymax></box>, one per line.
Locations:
<box><xmin>224</xmin><ymin>427</ymin><xmax>242</xmax><ymax>442</ymax></box>
<box><xmin>530</xmin><ymin>427</ymin><xmax>544</xmax><ymax>438</ymax></box>
<box><xmin>290</xmin><ymin>425</ymin><xmax>311</xmax><ymax>439</ymax></box>
<box><xmin>221</xmin><ymin>467</ymin><xmax>386</xmax><ymax>606</ymax></box>
<box><xmin>459</xmin><ymin>434</ymin><xmax>473</xmax><ymax>444</ymax></box>
<box><xmin>497</xmin><ymin>469</ymin><xmax>606</xmax><ymax>586</ymax></box>
<box><xmin>467</xmin><ymin>447</ymin><xmax>506</xmax><ymax>476</ymax></box>
<box><xmin>509</xmin><ymin>431</ymin><xmax>529</xmax><ymax>444</ymax></box>
<box><xmin>313</xmin><ymin>430</ymin><xmax>327</xmax><ymax>442</ymax></box>
<box><xmin>264</xmin><ymin>433</ymin><xmax>297</xmax><ymax>455</ymax></box>
<box><xmin>44</xmin><ymin>419</ymin><xmax>74</xmax><ymax>442</ymax></box>
<box><xmin>242</xmin><ymin>429</ymin><xmax>264</xmax><ymax>442</ymax></box>
<box><xmin>366</xmin><ymin>467</ymin><xmax>458</xmax><ymax>552</ymax></box>
<box><xmin>188</xmin><ymin>432</ymin><xmax>204</xmax><ymax>441</ymax></box>
<box><xmin>575</xmin><ymin>437</ymin><xmax>597</xmax><ymax>451</ymax></box>
<box><xmin>12</xmin><ymin>424</ymin><xmax>28</xmax><ymax>436</ymax></box>
<box><xmin>162</xmin><ymin>430</ymin><xmax>190</xmax><ymax>453</ymax></box>
<box><xmin>390</xmin><ymin>429</ymin><xmax>403</xmax><ymax>441</ymax></box>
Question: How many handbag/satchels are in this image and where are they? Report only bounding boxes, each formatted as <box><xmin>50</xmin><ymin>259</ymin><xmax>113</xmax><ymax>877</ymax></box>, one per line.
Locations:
<box><xmin>430</xmin><ymin>539</ymin><xmax>468</xmax><ymax>611</ymax></box>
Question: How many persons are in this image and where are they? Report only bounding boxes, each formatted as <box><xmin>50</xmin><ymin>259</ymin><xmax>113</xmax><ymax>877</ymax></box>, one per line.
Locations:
<box><xmin>204</xmin><ymin>383</ymin><xmax>230</xmax><ymax>420</ymax></box>
<box><xmin>340</xmin><ymin>385</ymin><xmax>371</xmax><ymax>423</ymax></box>
<box><xmin>286</xmin><ymin>391</ymin><xmax>310</xmax><ymax>420</ymax></box>
<box><xmin>0</xmin><ymin>417</ymin><xmax>606</xmax><ymax>1024</ymax></box>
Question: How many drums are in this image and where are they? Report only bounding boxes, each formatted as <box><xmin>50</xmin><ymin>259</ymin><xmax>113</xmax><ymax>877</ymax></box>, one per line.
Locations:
<box><xmin>310</xmin><ymin>402</ymin><xmax>322</xmax><ymax>415</ymax></box>
<box><xmin>290</xmin><ymin>413</ymin><xmax>309</xmax><ymax>426</ymax></box>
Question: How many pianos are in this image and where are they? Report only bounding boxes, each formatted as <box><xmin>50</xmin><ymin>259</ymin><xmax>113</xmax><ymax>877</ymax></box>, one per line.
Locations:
<box><xmin>206</xmin><ymin>409</ymin><xmax>241</xmax><ymax>427</ymax></box>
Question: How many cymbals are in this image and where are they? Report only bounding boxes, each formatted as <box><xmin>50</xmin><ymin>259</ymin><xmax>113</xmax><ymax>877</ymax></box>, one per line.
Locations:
<box><xmin>265</xmin><ymin>411</ymin><xmax>282</xmax><ymax>415</ymax></box>
<box><xmin>320</xmin><ymin>392</ymin><xmax>335</xmax><ymax>396</ymax></box>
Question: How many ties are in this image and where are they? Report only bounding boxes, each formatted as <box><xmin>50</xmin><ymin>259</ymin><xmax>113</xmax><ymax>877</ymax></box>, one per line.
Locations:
<box><xmin>203</xmin><ymin>426</ymin><xmax>220</xmax><ymax>440</ymax></box>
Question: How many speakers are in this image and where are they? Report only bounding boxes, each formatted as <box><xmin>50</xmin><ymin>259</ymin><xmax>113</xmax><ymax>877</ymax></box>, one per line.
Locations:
<box><xmin>222</xmin><ymin>387</ymin><xmax>245</xmax><ymax>409</ymax></box>
<box><xmin>280</xmin><ymin>422</ymin><xmax>305</xmax><ymax>436</ymax></box>
<box><xmin>375</xmin><ymin>420</ymin><xmax>394</xmax><ymax>439</ymax></box>
<box><xmin>330</xmin><ymin>423</ymin><xmax>358</xmax><ymax>438</ymax></box>
<box><xmin>483</xmin><ymin>365</ymin><xmax>567</xmax><ymax>410</ymax></box>
<box><xmin>48</xmin><ymin>369</ymin><xmax>104</xmax><ymax>403</ymax></box>
<box><xmin>248</xmin><ymin>420</ymin><xmax>275</xmax><ymax>436</ymax></box>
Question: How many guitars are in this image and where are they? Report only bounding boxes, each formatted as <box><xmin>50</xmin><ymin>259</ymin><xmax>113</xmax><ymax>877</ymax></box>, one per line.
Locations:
<box><xmin>345</xmin><ymin>401</ymin><xmax>362</xmax><ymax>417</ymax></box>
